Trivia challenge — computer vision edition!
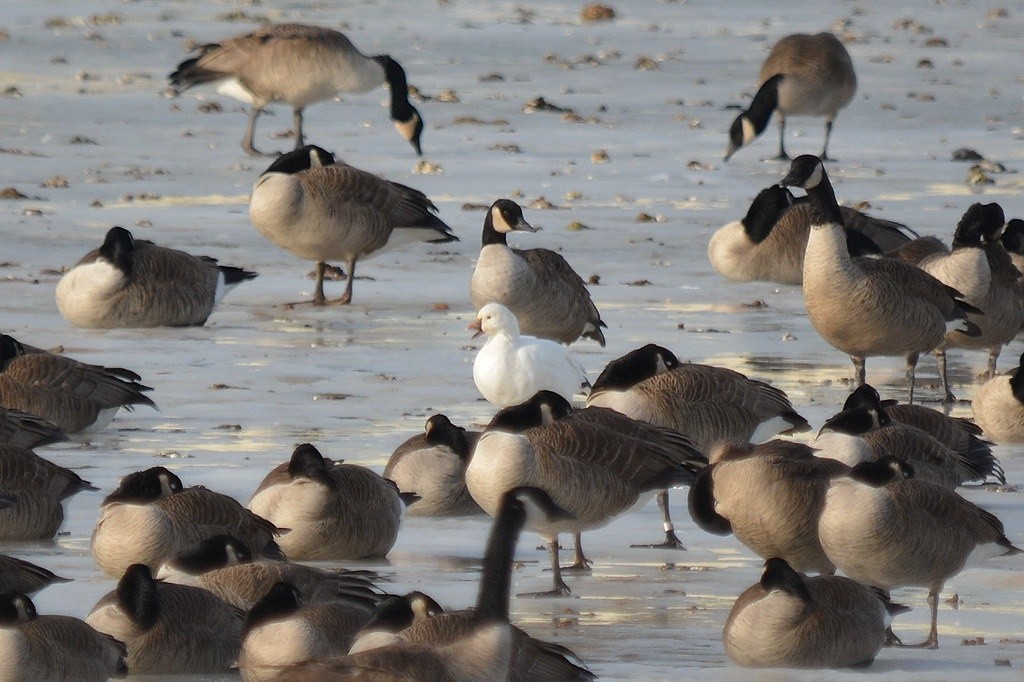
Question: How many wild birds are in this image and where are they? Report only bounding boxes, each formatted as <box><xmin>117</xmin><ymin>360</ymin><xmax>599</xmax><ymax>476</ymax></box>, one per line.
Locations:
<box><xmin>56</xmin><ymin>226</ymin><xmax>261</xmax><ymax>331</ymax></box>
<box><xmin>249</xmin><ymin>143</ymin><xmax>463</xmax><ymax>308</ymax></box>
<box><xmin>381</xmin><ymin>198</ymin><xmax>812</xmax><ymax>600</ymax></box>
<box><xmin>169</xmin><ymin>19</ymin><xmax>426</xmax><ymax>160</ymax></box>
<box><xmin>720</xmin><ymin>30</ymin><xmax>858</xmax><ymax>165</ymax></box>
<box><xmin>1</xmin><ymin>330</ymin><xmax>603</xmax><ymax>682</ymax></box>
<box><xmin>681</xmin><ymin>153</ymin><xmax>1024</xmax><ymax>668</ymax></box>
